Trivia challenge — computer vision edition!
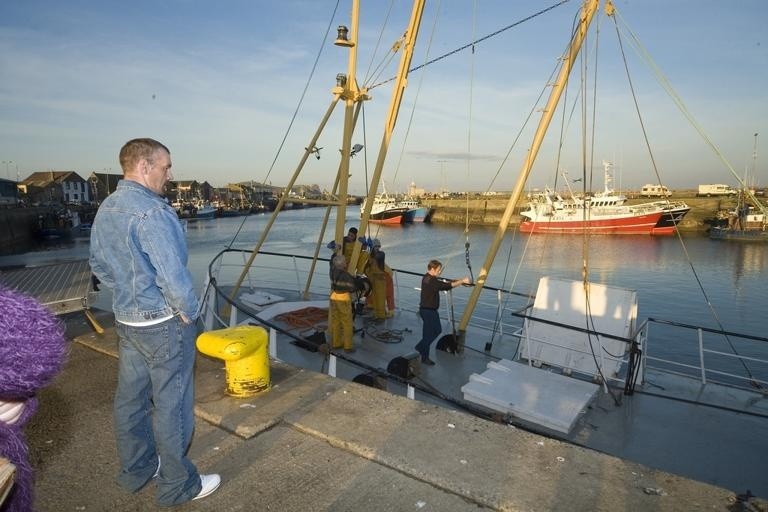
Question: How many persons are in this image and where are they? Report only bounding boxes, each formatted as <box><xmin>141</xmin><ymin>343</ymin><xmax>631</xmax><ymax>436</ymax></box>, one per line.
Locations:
<box><xmin>327</xmin><ymin>228</ymin><xmax>395</xmax><ymax>353</ymax></box>
<box><xmin>415</xmin><ymin>260</ymin><xmax>471</xmax><ymax>365</ymax></box>
<box><xmin>88</xmin><ymin>138</ymin><xmax>222</xmax><ymax>507</ymax></box>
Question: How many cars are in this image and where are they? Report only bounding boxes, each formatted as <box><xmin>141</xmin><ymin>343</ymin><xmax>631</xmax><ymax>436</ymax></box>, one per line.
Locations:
<box><xmin>755</xmin><ymin>190</ymin><xmax>766</xmax><ymax>197</ymax></box>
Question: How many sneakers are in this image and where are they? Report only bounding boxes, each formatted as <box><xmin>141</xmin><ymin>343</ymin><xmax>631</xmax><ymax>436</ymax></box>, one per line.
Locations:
<box><xmin>190</xmin><ymin>474</ymin><xmax>221</xmax><ymax>500</ymax></box>
<box><xmin>415</xmin><ymin>342</ymin><xmax>435</xmax><ymax>365</ymax></box>
<box><xmin>152</xmin><ymin>455</ymin><xmax>161</xmax><ymax>478</ymax></box>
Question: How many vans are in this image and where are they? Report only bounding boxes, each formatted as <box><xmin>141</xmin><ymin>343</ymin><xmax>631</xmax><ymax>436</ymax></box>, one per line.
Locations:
<box><xmin>698</xmin><ymin>184</ymin><xmax>736</xmax><ymax>198</ymax></box>
<box><xmin>641</xmin><ymin>183</ymin><xmax>672</xmax><ymax>199</ymax></box>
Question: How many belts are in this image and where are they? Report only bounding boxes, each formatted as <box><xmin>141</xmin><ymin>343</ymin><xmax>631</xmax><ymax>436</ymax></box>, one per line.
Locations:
<box><xmin>420</xmin><ymin>307</ymin><xmax>438</xmax><ymax>311</ymax></box>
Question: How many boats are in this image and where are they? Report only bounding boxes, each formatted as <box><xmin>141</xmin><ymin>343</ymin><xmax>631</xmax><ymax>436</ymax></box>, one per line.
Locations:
<box><xmin>360</xmin><ymin>171</ymin><xmax>431</xmax><ymax>224</ymax></box>
<box><xmin>32</xmin><ymin>198</ymin><xmax>97</xmax><ymax>239</ymax></box>
<box><xmin>706</xmin><ymin>131</ymin><xmax>768</xmax><ymax>239</ymax></box>
<box><xmin>193</xmin><ymin>1</ymin><xmax>768</xmax><ymax>502</ymax></box>
<box><xmin>172</xmin><ymin>183</ymin><xmax>266</xmax><ymax>217</ymax></box>
<box><xmin>518</xmin><ymin>159</ymin><xmax>692</xmax><ymax>240</ymax></box>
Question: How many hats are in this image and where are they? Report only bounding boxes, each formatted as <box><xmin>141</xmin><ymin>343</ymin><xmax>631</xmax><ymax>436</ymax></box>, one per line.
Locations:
<box><xmin>372</xmin><ymin>239</ymin><xmax>381</xmax><ymax>248</ymax></box>
